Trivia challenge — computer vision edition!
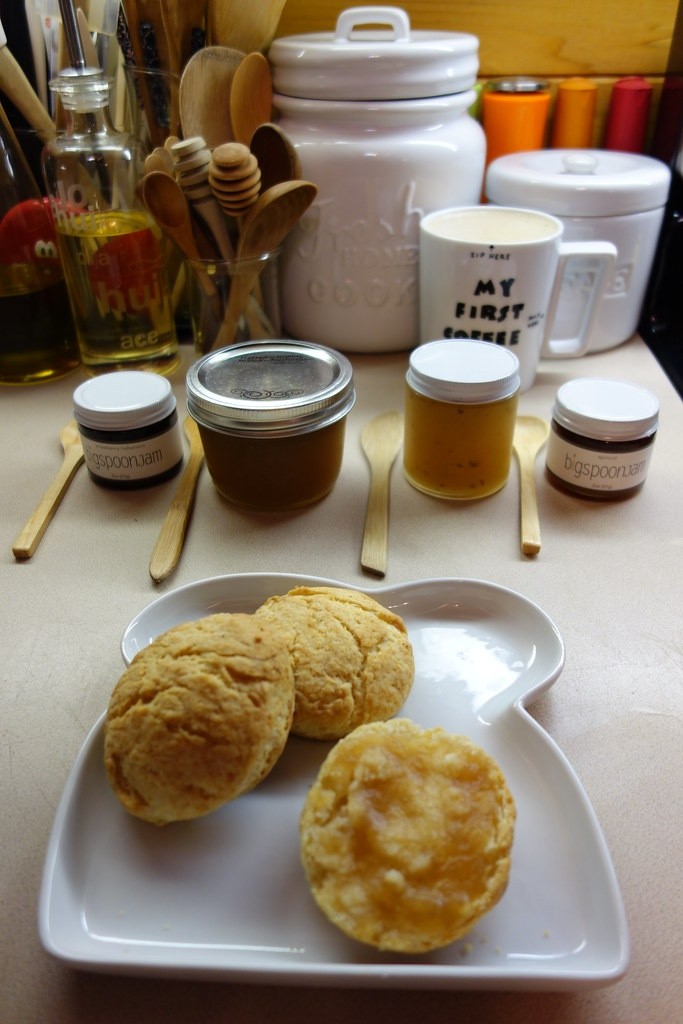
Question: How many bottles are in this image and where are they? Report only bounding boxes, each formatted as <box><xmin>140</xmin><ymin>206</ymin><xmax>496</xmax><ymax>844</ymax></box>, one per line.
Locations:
<box><xmin>261</xmin><ymin>5</ymin><xmax>490</xmax><ymax>353</ymax></box>
<box><xmin>42</xmin><ymin>66</ymin><xmax>182</xmax><ymax>379</ymax></box>
<box><xmin>182</xmin><ymin>337</ymin><xmax>358</xmax><ymax>513</ymax></box>
<box><xmin>402</xmin><ymin>338</ymin><xmax>523</xmax><ymax>502</ymax></box>
<box><xmin>480</xmin><ymin>76</ymin><xmax>552</xmax><ymax>208</ymax></box>
<box><xmin>484</xmin><ymin>145</ymin><xmax>671</xmax><ymax>359</ymax></box>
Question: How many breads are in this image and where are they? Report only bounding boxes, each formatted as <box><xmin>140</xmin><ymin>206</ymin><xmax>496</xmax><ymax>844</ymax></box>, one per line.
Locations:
<box><xmin>298</xmin><ymin>718</ymin><xmax>517</xmax><ymax>952</ymax></box>
<box><xmin>252</xmin><ymin>586</ymin><xmax>416</xmax><ymax>741</ymax></box>
<box><xmin>104</xmin><ymin>611</ymin><xmax>294</xmax><ymax>824</ymax></box>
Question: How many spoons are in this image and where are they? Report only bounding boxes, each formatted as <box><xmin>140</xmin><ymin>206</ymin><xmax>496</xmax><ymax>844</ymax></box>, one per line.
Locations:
<box><xmin>360</xmin><ymin>410</ymin><xmax>407</xmax><ymax>579</ymax></box>
<box><xmin>11</xmin><ymin>421</ymin><xmax>85</xmax><ymax>559</ymax></box>
<box><xmin>134</xmin><ymin>122</ymin><xmax>321</xmax><ymax>349</ymax></box>
<box><xmin>513</xmin><ymin>415</ymin><xmax>549</xmax><ymax>555</ymax></box>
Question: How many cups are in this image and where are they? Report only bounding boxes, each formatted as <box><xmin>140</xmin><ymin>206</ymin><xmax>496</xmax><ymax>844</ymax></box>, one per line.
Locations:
<box><xmin>414</xmin><ymin>205</ymin><xmax>617</xmax><ymax>391</ymax></box>
<box><xmin>182</xmin><ymin>250</ymin><xmax>280</xmax><ymax>353</ymax></box>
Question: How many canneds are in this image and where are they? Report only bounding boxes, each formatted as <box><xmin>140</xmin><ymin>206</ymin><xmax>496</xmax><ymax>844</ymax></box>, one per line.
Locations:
<box><xmin>72</xmin><ymin>370</ymin><xmax>185</xmax><ymax>490</ymax></box>
<box><xmin>185</xmin><ymin>339</ymin><xmax>356</xmax><ymax>513</ymax></box>
<box><xmin>544</xmin><ymin>378</ymin><xmax>660</xmax><ymax>501</ymax></box>
<box><xmin>403</xmin><ymin>338</ymin><xmax>521</xmax><ymax>501</ymax></box>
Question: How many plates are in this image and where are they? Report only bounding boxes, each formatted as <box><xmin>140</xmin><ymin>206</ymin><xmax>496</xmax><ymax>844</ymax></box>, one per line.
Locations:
<box><xmin>39</xmin><ymin>570</ymin><xmax>632</xmax><ymax>994</ymax></box>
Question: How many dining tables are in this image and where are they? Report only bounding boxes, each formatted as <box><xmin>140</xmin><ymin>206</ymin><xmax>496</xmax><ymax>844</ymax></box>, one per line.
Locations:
<box><xmin>0</xmin><ymin>306</ymin><xmax>683</xmax><ymax>1024</ymax></box>
